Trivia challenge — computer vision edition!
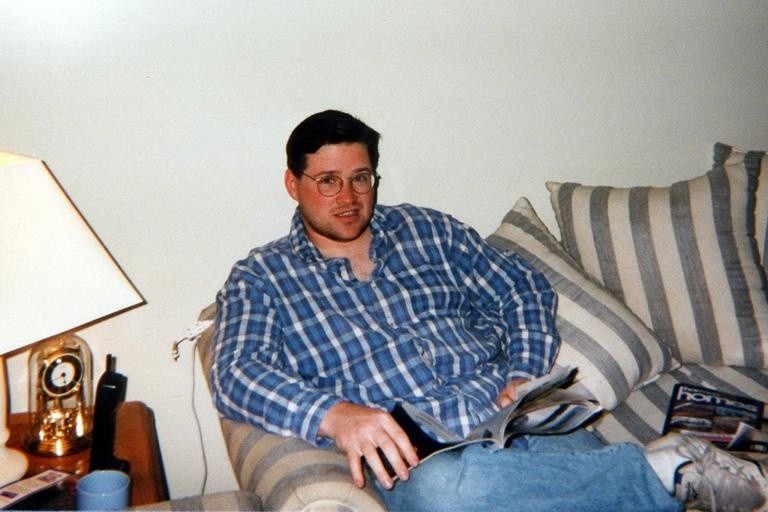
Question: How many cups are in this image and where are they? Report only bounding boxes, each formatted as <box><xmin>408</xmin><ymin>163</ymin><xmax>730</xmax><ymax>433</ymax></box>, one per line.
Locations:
<box><xmin>74</xmin><ymin>467</ymin><xmax>130</xmax><ymax>512</ymax></box>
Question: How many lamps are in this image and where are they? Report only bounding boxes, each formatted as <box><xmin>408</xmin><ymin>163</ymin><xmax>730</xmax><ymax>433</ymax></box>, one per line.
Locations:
<box><xmin>0</xmin><ymin>149</ymin><xmax>147</xmax><ymax>487</ymax></box>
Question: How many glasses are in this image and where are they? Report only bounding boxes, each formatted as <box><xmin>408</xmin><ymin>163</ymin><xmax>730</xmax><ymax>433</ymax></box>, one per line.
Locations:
<box><xmin>303</xmin><ymin>172</ymin><xmax>381</xmax><ymax>197</ymax></box>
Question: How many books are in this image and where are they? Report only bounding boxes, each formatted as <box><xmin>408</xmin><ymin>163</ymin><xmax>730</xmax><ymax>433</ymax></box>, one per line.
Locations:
<box><xmin>724</xmin><ymin>419</ymin><xmax>767</xmax><ymax>480</ymax></box>
<box><xmin>661</xmin><ymin>381</ymin><xmax>767</xmax><ymax>452</ymax></box>
<box><xmin>377</xmin><ymin>362</ymin><xmax>606</xmax><ymax>487</ymax></box>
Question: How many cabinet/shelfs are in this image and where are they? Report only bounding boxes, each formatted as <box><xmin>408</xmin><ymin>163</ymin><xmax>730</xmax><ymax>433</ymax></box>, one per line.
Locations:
<box><xmin>0</xmin><ymin>402</ymin><xmax>173</xmax><ymax>512</ymax></box>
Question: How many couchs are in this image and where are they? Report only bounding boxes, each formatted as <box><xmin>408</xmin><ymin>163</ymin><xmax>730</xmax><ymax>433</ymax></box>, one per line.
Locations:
<box><xmin>193</xmin><ymin>237</ymin><xmax>768</xmax><ymax>512</ymax></box>
<box><xmin>108</xmin><ymin>489</ymin><xmax>262</xmax><ymax>511</ymax></box>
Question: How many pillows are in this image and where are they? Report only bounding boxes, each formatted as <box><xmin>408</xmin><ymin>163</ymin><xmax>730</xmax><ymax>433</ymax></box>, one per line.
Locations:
<box><xmin>479</xmin><ymin>193</ymin><xmax>679</xmax><ymax>412</ymax></box>
<box><xmin>714</xmin><ymin>142</ymin><xmax>767</xmax><ymax>279</ymax></box>
<box><xmin>544</xmin><ymin>161</ymin><xmax>767</xmax><ymax>370</ymax></box>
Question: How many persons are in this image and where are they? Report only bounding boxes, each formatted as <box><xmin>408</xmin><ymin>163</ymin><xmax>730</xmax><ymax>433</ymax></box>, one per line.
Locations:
<box><xmin>207</xmin><ymin>105</ymin><xmax>768</xmax><ymax>512</ymax></box>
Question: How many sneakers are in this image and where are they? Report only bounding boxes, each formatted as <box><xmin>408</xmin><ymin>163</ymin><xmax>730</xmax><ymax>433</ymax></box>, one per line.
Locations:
<box><xmin>644</xmin><ymin>431</ymin><xmax>768</xmax><ymax>512</ymax></box>
